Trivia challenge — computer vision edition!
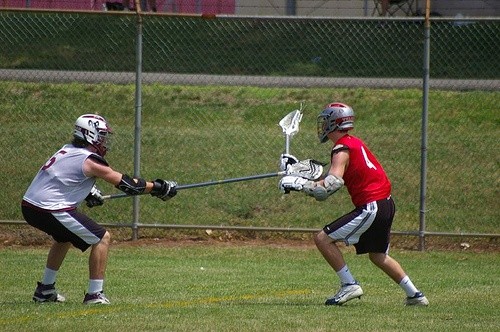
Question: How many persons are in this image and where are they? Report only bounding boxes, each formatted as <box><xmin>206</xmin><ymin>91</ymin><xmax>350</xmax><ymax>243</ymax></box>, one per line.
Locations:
<box><xmin>278</xmin><ymin>102</ymin><xmax>431</xmax><ymax>309</ymax></box>
<box><xmin>21</xmin><ymin>112</ymin><xmax>178</xmax><ymax>305</ymax></box>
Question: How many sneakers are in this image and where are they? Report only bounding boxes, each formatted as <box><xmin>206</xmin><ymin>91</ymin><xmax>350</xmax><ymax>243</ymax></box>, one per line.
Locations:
<box><xmin>405</xmin><ymin>291</ymin><xmax>430</xmax><ymax>308</ymax></box>
<box><xmin>32</xmin><ymin>282</ymin><xmax>66</xmax><ymax>304</ymax></box>
<box><xmin>323</xmin><ymin>280</ymin><xmax>365</xmax><ymax>306</ymax></box>
<box><xmin>82</xmin><ymin>293</ymin><xmax>111</xmax><ymax>305</ymax></box>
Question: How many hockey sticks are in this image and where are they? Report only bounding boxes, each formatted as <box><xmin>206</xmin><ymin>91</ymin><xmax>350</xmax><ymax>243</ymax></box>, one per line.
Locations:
<box><xmin>101</xmin><ymin>159</ymin><xmax>328</xmax><ymax>200</ymax></box>
<box><xmin>278</xmin><ymin>109</ymin><xmax>303</xmax><ymax>195</ymax></box>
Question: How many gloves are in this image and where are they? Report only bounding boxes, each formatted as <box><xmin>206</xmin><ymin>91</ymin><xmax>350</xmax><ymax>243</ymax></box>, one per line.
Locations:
<box><xmin>279</xmin><ymin>153</ymin><xmax>299</xmax><ymax>172</ymax></box>
<box><xmin>277</xmin><ymin>175</ymin><xmax>309</xmax><ymax>195</ymax></box>
<box><xmin>84</xmin><ymin>186</ymin><xmax>104</xmax><ymax>208</ymax></box>
<box><xmin>152</xmin><ymin>179</ymin><xmax>178</xmax><ymax>202</ymax></box>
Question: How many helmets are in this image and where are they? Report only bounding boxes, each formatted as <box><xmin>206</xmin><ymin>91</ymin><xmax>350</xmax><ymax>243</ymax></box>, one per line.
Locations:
<box><xmin>316</xmin><ymin>101</ymin><xmax>356</xmax><ymax>143</ymax></box>
<box><xmin>72</xmin><ymin>113</ymin><xmax>113</xmax><ymax>157</ymax></box>
